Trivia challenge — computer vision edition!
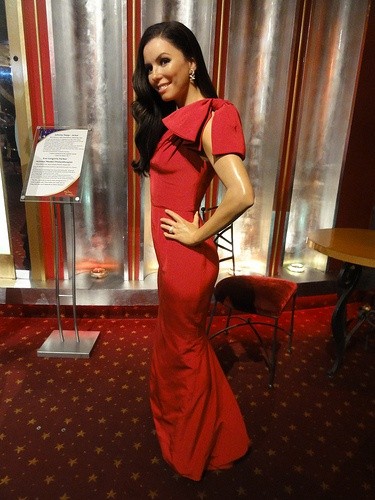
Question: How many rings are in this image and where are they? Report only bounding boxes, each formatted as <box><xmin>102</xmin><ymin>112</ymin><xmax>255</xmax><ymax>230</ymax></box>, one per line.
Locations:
<box><xmin>168</xmin><ymin>226</ymin><xmax>174</xmax><ymax>233</ymax></box>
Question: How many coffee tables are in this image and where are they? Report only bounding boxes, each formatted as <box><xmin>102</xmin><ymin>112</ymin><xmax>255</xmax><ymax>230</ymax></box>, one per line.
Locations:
<box><xmin>305</xmin><ymin>228</ymin><xmax>375</xmax><ymax>376</ymax></box>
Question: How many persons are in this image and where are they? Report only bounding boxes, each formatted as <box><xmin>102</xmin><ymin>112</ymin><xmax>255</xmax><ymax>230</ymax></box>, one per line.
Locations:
<box><xmin>127</xmin><ymin>18</ymin><xmax>255</xmax><ymax>484</ymax></box>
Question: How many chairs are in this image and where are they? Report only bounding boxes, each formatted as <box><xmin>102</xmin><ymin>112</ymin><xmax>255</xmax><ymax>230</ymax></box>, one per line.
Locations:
<box><xmin>200</xmin><ymin>205</ymin><xmax>297</xmax><ymax>385</ymax></box>
